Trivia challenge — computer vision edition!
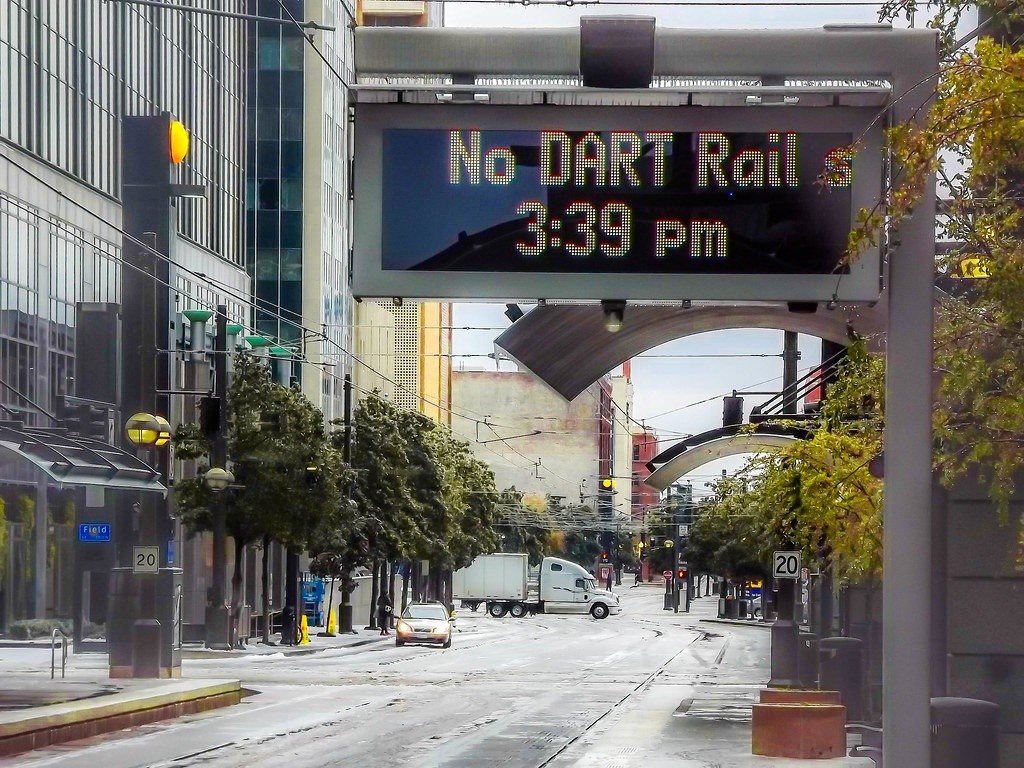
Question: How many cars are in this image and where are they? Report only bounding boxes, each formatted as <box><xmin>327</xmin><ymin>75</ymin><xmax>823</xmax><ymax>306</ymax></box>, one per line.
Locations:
<box><xmin>392</xmin><ymin>600</ymin><xmax>456</xmax><ymax>648</ymax></box>
<box><xmin>747</xmin><ymin>595</ymin><xmax>763</xmax><ymax>618</ymax></box>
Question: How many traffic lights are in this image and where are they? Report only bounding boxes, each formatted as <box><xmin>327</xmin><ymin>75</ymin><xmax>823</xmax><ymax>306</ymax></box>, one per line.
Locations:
<box><xmin>678</xmin><ymin>570</ymin><xmax>686</xmax><ymax>579</ymax></box>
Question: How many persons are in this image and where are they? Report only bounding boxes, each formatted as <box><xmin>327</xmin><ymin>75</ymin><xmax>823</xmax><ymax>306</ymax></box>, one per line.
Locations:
<box><xmin>606</xmin><ymin>573</ymin><xmax>612</xmax><ymax>592</ymax></box>
<box><xmin>377</xmin><ymin>588</ymin><xmax>391</xmax><ymax>635</ymax></box>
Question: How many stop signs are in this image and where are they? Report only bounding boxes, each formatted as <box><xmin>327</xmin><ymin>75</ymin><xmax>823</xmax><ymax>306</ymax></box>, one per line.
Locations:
<box><xmin>664</xmin><ymin>571</ymin><xmax>673</xmax><ymax>579</ymax></box>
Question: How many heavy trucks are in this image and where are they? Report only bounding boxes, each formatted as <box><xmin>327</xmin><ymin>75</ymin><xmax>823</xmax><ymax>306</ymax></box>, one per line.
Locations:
<box><xmin>451</xmin><ymin>553</ymin><xmax>621</xmax><ymax>619</ymax></box>
